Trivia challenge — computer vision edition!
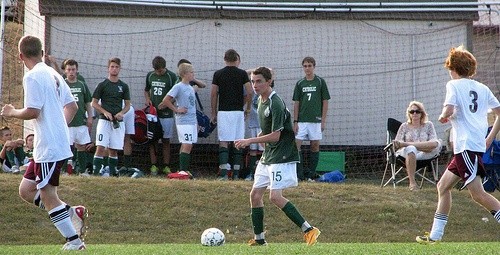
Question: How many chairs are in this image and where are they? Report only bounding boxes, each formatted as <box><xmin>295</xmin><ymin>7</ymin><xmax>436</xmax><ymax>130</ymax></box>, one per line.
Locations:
<box><xmin>381</xmin><ymin>117</ymin><xmax>439</xmax><ymax>190</ymax></box>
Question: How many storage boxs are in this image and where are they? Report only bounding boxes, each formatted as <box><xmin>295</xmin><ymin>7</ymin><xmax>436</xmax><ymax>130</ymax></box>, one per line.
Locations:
<box><xmin>315</xmin><ymin>151</ymin><xmax>346</xmax><ymax>176</ymax></box>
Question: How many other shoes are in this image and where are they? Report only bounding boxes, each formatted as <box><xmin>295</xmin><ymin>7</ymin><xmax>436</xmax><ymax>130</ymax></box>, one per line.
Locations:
<box><xmin>298</xmin><ymin>174</ymin><xmax>305</xmax><ymax>181</ymax></box>
<box><xmin>308</xmin><ymin>173</ymin><xmax>315</xmax><ymax>182</ymax></box>
<box><xmin>216</xmin><ymin>171</ymin><xmax>228</xmax><ymax>180</ymax></box>
<box><xmin>245</xmin><ymin>174</ymin><xmax>254</xmax><ymax>181</ymax></box>
<box><xmin>232</xmin><ymin>174</ymin><xmax>239</xmax><ymax>181</ymax></box>
<box><xmin>393</xmin><ymin>140</ymin><xmax>400</xmax><ymax>152</ymax></box>
<box><xmin>409</xmin><ymin>183</ymin><xmax>420</xmax><ymax>191</ymax></box>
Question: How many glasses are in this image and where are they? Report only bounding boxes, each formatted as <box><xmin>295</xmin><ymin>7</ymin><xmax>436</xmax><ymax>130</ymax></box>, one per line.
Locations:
<box><xmin>409</xmin><ymin>110</ymin><xmax>421</xmax><ymax>114</ymax></box>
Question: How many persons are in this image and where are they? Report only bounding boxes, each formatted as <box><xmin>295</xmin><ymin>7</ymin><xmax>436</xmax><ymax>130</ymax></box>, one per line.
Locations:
<box><xmin>0</xmin><ymin>126</ymin><xmax>35</xmax><ymax>174</ymax></box>
<box><xmin>0</xmin><ymin>35</ymin><xmax>90</xmax><ymax>251</ymax></box>
<box><xmin>391</xmin><ymin>101</ymin><xmax>443</xmax><ymax>191</ymax></box>
<box><xmin>414</xmin><ymin>45</ymin><xmax>500</xmax><ymax>244</ymax></box>
<box><xmin>292</xmin><ymin>56</ymin><xmax>331</xmax><ymax>181</ymax></box>
<box><xmin>205</xmin><ymin>47</ymin><xmax>274</xmax><ymax>182</ymax></box>
<box><xmin>49</xmin><ymin>57</ymin><xmax>207</xmax><ymax>182</ymax></box>
<box><xmin>231</xmin><ymin>65</ymin><xmax>322</xmax><ymax>246</ymax></box>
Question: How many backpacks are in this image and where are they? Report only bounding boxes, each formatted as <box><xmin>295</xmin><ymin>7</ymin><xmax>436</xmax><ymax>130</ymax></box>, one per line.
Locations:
<box><xmin>128</xmin><ymin>104</ymin><xmax>164</xmax><ymax>146</ymax></box>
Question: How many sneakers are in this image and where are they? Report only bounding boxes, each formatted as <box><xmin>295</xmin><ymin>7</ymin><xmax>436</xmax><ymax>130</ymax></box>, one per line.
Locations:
<box><xmin>416</xmin><ymin>231</ymin><xmax>436</xmax><ymax>245</ymax></box>
<box><xmin>70</xmin><ymin>205</ymin><xmax>89</xmax><ymax>243</ymax></box>
<box><xmin>162</xmin><ymin>165</ymin><xmax>171</xmax><ymax>175</ymax></box>
<box><xmin>248</xmin><ymin>239</ymin><xmax>268</xmax><ymax>246</ymax></box>
<box><xmin>3</xmin><ymin>165</ymin><xmax>12</xmax><ymax>173</ymax></box>
<box><xmin>12</xmin><ymin>165</ymin><xmax>20</xmax><ymax>174</ymax></box>
<box><xmin>131</xmin><ymin>170</ymin><xmax>144</xmax><ymax>178</ymax></box>
<box><xmin>303</xmin><ymin>226</ymin><xmax>320</xmax><ymax>246</ymax></box>
<box><xmin>150</xmin><ymin>166</ymin><xmax>158</xmax><ymax>175</ymax></box>
<box><xmin>61</xmin><ymin>241</ymin><xmax>87</xmax><ymax>251</ymax></box>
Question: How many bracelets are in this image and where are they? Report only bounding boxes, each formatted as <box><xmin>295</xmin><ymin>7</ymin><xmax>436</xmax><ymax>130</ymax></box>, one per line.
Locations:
<box><xmin>293</xmin><ymin>120</ymin><xmax>298</xmax><ymax>123</ymax></box>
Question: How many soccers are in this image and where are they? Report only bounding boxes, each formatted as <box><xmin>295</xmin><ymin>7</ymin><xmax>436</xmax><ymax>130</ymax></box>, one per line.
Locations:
<box><xmin>201</xmin><ymin>228</ymin><xmax>225</xmax><ymax>246</ymax></box>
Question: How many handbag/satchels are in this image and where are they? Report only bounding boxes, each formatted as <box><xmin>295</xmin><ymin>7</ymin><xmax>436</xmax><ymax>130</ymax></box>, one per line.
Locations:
<box><xmin>320</xmin><ymin>170</ymin><xmax>347</xmax><ymax>183</ymax></box>
<box><xmin>196</xmin><ymin>110</ymin><xmax>217</xmax><ymax>139</ymax></box>
